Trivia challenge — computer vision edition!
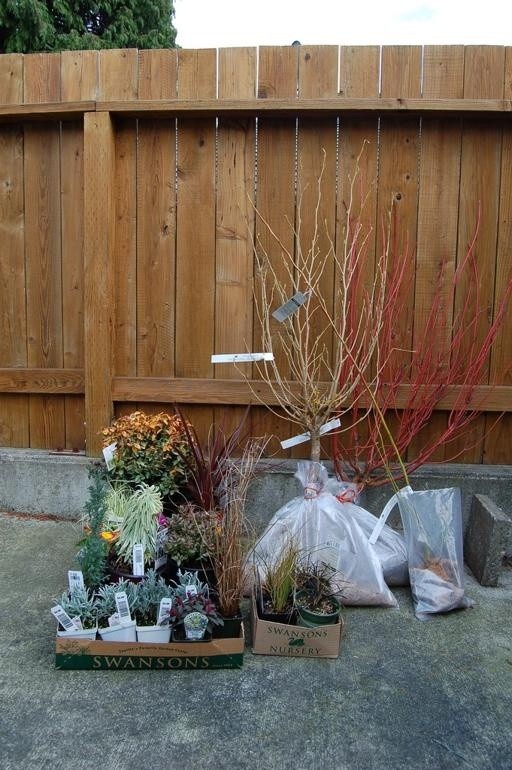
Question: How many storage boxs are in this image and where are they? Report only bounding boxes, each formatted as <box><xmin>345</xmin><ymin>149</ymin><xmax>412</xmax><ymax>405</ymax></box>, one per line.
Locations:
<box><xmin>250</xmin><ymin>581</ymin><xmax>345</xmax><ymax>659</ymax></box>
<box><xmin>55</xmin><ymin>584</ymin><xmax>245</xmax><ymax>667</ymax></box>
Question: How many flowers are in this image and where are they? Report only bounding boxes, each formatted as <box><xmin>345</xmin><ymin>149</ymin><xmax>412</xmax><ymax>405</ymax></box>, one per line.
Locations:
<box><xmin>96</xmin><ymin>412</ymin><xmax>198</xmax><ymax>451</ymax></box>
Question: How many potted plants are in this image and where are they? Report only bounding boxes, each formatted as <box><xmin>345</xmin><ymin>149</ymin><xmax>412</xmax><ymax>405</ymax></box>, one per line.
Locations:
<box><xmin>96</xmin><ymin>576</ymin><xmax>136</xmax><ymax>641</ymax></box>
<box><xmin>176</xmin><ymin>571</ymin><xmax>216</xmax><ymax>643</ymax></box>
<box><xmin>253</xmin><ymin>543</ymin><xmax>298</xmax><ymax>622</ymax></box>
<box><xmin>135</xmin><ymin>568</ymin><xmax>173</xmax><ymax>643</ymax></box>
<box><xmin>105</xmin><ymin>483</ymin><xmax>168</xmax><ymax>581</ymax></box>
<box><xmin>52</xmin><ymin>585</ymin><xmax>97</xmax><ymax>639</ymax></box>
<box><xmin>169</xmin><ymin>505</ymin><xmax>226</xmax><ymax>581</ymax></box>
<box><xmin>293</xmin><ymin>560</ymin><xmax>344</xmax><ymax>625</ymax></box>
